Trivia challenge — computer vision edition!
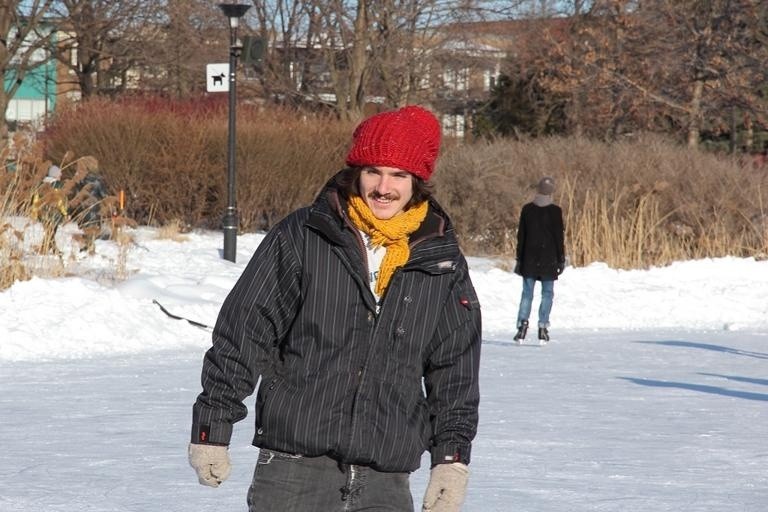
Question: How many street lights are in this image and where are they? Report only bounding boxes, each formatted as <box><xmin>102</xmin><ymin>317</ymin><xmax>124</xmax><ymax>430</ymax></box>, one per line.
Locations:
<box><xmin>216</xmin><ymin>3</ymin><xmax>267</xmax><ymax>262</ymax></box>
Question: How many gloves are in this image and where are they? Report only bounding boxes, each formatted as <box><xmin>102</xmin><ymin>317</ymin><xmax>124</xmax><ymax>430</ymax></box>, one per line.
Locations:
<box><xmin>188</xmin><ymin>443</ymin><xmax>231</xmax><ymax>484</ymax></box>
<box><xmin>423</xmin><ymin>461</ymin><xmax>469</xmax><ymax>510</ymax></box>
<box><xmin>556</xmin><ymin>262</ymin><xmax>565</xmax><ymax>275</ymax></box>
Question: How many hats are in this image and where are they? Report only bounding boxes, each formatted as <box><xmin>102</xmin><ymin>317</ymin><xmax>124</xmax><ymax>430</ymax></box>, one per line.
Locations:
<box><xmin>347</xmin><ymin>104</ymin><xmax>443</xmax><ymax>180</ymax></box>
<box><xmin>540</xmin><ymin>177</ymin><xmax>557</xmax><ymax>199</ymax></box>
<box><xmin>48</xmin><ymin>166</ymin><xmax>63</xmax><ymax>179</ymax></box>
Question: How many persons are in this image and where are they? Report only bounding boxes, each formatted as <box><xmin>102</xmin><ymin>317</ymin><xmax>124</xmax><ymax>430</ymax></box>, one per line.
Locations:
<box><xmin>185</xmin><ymin>107</ymin><xmax>483</xmax><ymax>512</ymax></box>
<box><xmin>33</xmin><ymin>163</ymin><xmax>68</xmax><ymax>259</ymax></box>
<box><xmin>71</xmin><ymin>165</ymin><xmax>115</xmax><ymax>254</ymax></box>
<box><xmin>512</xmin><ymin>175</ymin><xmax>567</xmax><ymax>343</ymax></box>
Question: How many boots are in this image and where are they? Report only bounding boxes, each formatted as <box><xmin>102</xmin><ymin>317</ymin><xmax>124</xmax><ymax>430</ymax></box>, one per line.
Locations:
<box><xmin>539</xmin><ymin>327</ymin><xmax>550</xmax><ymax>341</ymax></box>
<box><xmin>514</xmin><ymin>320</ymin><xmax>529</xmax><ymax>339</ymax></box>
